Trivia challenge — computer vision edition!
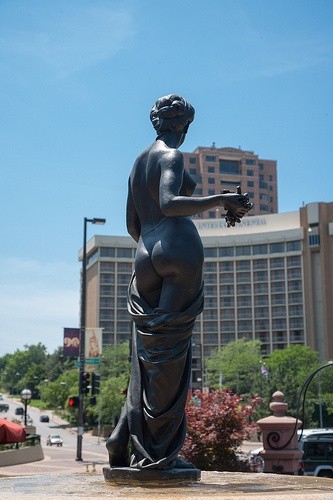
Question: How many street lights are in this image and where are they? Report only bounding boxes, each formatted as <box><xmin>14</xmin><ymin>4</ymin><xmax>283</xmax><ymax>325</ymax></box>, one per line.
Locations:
<box><xmin>20</xmin><ymin>388</ymin><xmax>32</xmax><ymax>426</ymax></box>
<box><xmin>74</xmin><ymin>216</ymin><xmax>106</xmax><ymax>462</ymax></box>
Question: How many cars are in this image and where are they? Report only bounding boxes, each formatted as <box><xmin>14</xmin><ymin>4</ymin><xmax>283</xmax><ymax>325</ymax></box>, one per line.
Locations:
<box><xmin>46</xmin><ymin>434</ymin><xmax>63</xmax><ymax>447</ymax></box>
<box><xmin>40</xmin><ymin>415</ymin><xmax>49</xmax><ymax>422</ymax></box>
<box><xmin>249</xmin><ymin>428</ymin><xmax>333</xmax><ymax>478</ymax></box>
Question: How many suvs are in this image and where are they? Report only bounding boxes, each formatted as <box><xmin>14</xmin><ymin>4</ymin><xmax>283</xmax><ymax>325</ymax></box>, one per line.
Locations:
<box><xmin>15</xmin><ymin>408</ymin><xmax>24</xmax><ymax>415</ymax></box>
<box><xmin>0</xmin><ymin>404</ymin><xmax>10</xmax><ymax>412</ymax></box>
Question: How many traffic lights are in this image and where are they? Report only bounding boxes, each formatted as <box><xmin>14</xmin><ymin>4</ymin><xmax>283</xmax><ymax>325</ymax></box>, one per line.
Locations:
<box><xmin>79</xmin><ymin>371</ymin><xmax>90</xmax><ymax>395</ymax></box>
<box><xmin>91</xmin><ymin>372</ymin><xmax>101</xmax><ymax>394</ymax></box>
<box><xmin>69</xmin><ymin>397</ymin><xmax>79</xmax><ymax>407</ymax></box>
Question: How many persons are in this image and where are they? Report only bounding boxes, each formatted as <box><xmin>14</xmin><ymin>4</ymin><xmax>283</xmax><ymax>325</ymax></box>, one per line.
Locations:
<box><xmin>106</xmin><ymin>94</ymin><xmax>253</xmax><ymax>468</ymax></box>
